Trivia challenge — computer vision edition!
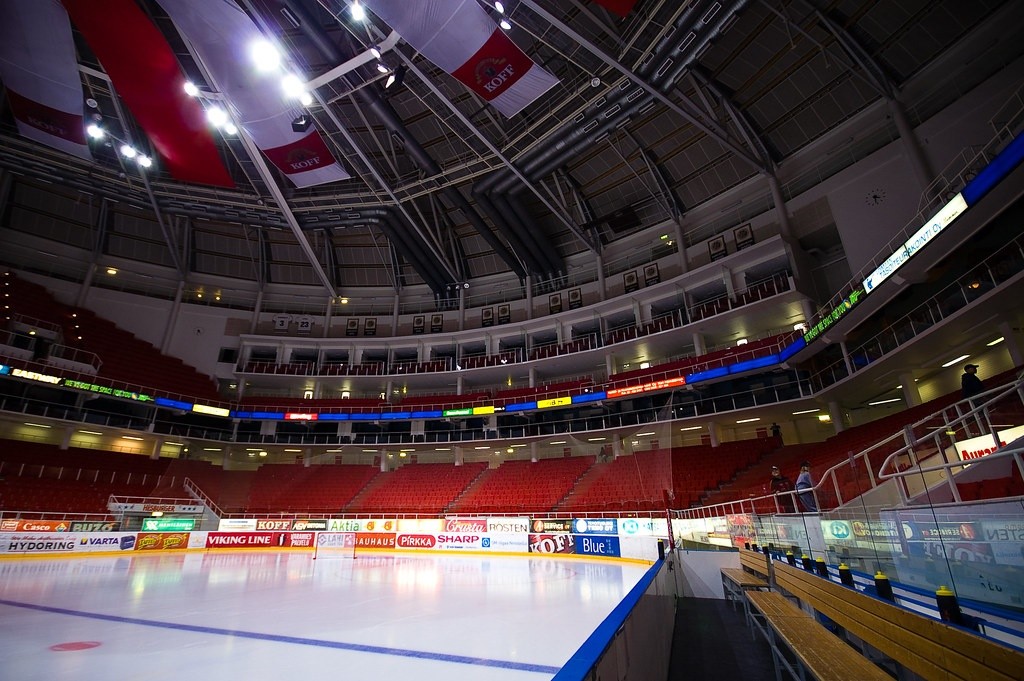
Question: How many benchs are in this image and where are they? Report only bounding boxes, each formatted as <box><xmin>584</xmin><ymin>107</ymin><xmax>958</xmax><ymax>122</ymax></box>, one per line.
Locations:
<box><xmin>720</xmin><ymin>546</ymin><xmax>772</xmax><ymax>627</ymax></box>
<box><xmin>744</xmin><ymin>558</ymin><xmax>1024</xmax><ymax>681</ymax></box>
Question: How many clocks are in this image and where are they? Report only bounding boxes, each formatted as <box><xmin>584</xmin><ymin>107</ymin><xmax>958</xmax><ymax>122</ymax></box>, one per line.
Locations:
<box><xmin>866</xmin><ymin>186</ymin><xmax>888</xmax><ymax>208</ymax></box>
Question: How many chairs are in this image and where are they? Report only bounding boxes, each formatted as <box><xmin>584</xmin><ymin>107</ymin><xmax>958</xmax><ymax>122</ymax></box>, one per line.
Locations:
<box><xmin>0</xmin><ymin>364</ymin><xmax>1024</xmax><ymax>520</ymax></box>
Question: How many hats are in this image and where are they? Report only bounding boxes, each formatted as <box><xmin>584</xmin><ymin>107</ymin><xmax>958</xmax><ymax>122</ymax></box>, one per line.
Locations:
<box><xmin>771</xmin><ymin>465</ymin><xmax>780</xmax><ymax>472</ymax></box>
<box><xmin>800</xmin><ymin>461</ymin><xmax>809</xmax><ymax>467</ymax></box>
<box><xmin>964</xmin><ymin>364</ymin><xmax>979</xmax><ymax>372</ymax></box>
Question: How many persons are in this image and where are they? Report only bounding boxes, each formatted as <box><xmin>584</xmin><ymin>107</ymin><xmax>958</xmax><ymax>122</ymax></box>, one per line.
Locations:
<box><xmin>770</xmin><ymin>422</ymin><xmax>781</xmax><ymax>436</ymax></box>
<box><xmin>598</xmin><ymin>446</ymin><xmax>609</xmax><ymax>463</ymax></box>
<box><xmin>796</xmin><ymin>461</ymin><xmax>818</xmax><ymax>512</ymax></box>
<box><xmin>770</xmin><ymin>464</ymin><xmax>795</xmax><ymax>513</ymax></box>
<box><xmin>961</xmin><ymin>364</ymin><xmax>984</xmax><ymax>420</ymax></box>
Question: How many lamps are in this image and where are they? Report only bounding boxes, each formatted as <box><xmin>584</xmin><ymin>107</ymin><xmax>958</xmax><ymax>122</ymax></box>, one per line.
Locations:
<box><xmin>355</xmin><ymin>6</ymin><xmax>406</xmax><ymax>87</ymax></box>
<box><xmin>292</xmin><ymin>114</ymin><xmax>311</xmax><ymax>132</ymax></box>
<box><xmin>185</xmin><ymin>81</ymin><xmax>237</xmax><ymax>134</ymax></box>
<box><xmin>90</xmin><ymin>127</ymin><xmax>152</xmax><ymax>166</ymax></box>
<box><xmin>496</xmin><ymin>1</ymin><xmax>512</xmax><ymax>31</ymax></box>
